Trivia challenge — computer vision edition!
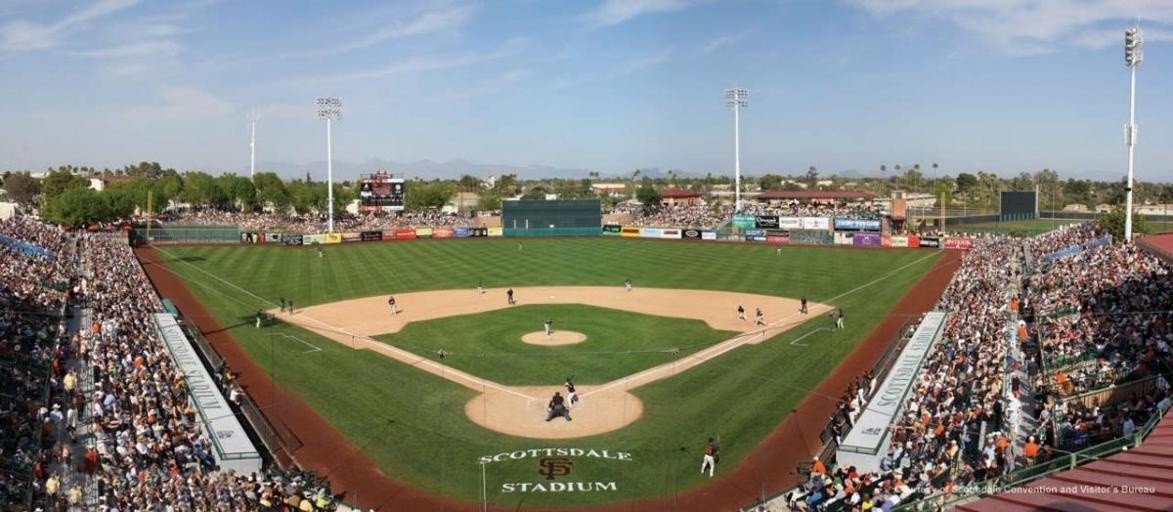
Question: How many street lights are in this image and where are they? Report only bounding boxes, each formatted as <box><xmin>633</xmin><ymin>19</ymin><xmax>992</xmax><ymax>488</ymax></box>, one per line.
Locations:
<box><xmin>315</xmin><ymin>96</ymin><xmax>343</xmax><ymax>234</ymax></box>
<box><xmin>1124</xmin><ymin>26</ymin><xmax>1145</xmax><ymax>244</ymax></box>
<box><xmin>723</xmin><ymin>86</ymin><xmax>750</xmax><ymax>216</ymax></box>
